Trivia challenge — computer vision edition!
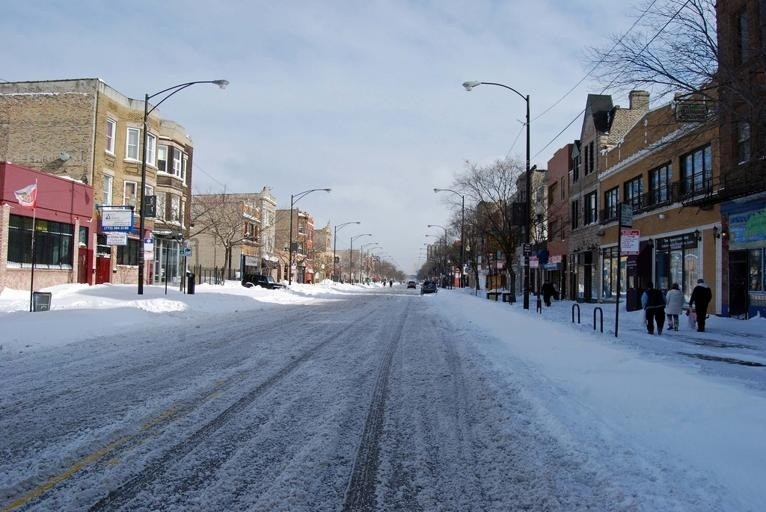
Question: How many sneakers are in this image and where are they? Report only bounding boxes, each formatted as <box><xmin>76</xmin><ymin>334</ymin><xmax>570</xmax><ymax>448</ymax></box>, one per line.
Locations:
<box><xmin>674</xmin><ymin>327</ymin><xmax>679</xmax><ymax>331</ymax></box>
<box><xmin>667</xmin><ymin>326</ymin><xmax>673</xmax><ymax>330</ymax></box>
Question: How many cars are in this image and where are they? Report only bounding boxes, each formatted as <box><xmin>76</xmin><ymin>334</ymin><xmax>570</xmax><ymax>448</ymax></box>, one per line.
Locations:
<box><xmin>415</xmin><ymin>281</ymin><xmax>419</xmax><ymax>285</ymax></box>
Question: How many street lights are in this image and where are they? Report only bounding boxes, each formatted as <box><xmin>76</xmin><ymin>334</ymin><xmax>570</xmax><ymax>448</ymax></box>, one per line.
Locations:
<box><xmin>136</xmin><ymin>78</ymin><xmax>231</xmax><ymax>295</ymax></box>
<box><xmin>350</xmin><ymin>232</ymin><xmax>401</xmax><ymax>284</ymax></box>
<box><xmin>463</xmin><ymin>79</ymin><xmax>531</xmax><ymax>311</ymax></box>
<box><xmin>332</xmin><ymin>220</ymin><xmax>361</xmax><ymax>281</ymax></box>
<box><xmin>417</xmin><ymin>223</ymin><xmax>448</xmax><ymax>285</ymax></box>
<box><xmin>286</xmin><ymin>188</ymin><xmax>331</xmax><ymax>285</ymax></box>
<box><xmin>434</xmin><ymin>188</ymin><xmax>466</xmax><ymax>288</ymax></box>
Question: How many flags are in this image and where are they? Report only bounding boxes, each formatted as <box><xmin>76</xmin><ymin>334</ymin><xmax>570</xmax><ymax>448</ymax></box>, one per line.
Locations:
<box><xmin>14</xmin><ymin>183</ymin><xmax>37</xmax><ymax>207</ymax></box>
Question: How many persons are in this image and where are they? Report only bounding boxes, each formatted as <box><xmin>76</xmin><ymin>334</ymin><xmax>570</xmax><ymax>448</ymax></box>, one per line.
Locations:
<box><xmin>689</xmin><ymin>279</ymin><xmax>712</xmax><ymax>332</ymax></box>
<box><xmin>642</xmin><ymin>283</ymin><xmax>667</xmax><ymax>335</ymax></box>
<box><xmin>541</xmin><ymin>280</ymin><xmax>555</xmax><ymax>306</ymax></box>
<box><xmin>665</xmin><ymin>283</ymin><xmax>686</xmax><ymax>331</ymax></box>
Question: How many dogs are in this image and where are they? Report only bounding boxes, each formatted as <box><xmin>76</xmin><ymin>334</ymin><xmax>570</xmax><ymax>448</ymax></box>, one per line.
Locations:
<box><xmin>685</xmin><ymin>307</ymin><xmax>698</xmax><ymax>329</ymax></box>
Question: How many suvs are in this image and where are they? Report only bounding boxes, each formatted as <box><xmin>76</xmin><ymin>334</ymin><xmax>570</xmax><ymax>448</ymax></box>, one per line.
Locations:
<box><xmin>421</xmin><ymin>281</ymin><xmax>438</xmax><ymax>294</ymax></box>
<box><xmin>149</xmin><ymin>260</ymin><xmax>165</xmax><ymax>280</ymax></box>
<box><xmin>242</xmin><ymin>272</ymin><xmax>287</xmax><ymax>290</ymax></box>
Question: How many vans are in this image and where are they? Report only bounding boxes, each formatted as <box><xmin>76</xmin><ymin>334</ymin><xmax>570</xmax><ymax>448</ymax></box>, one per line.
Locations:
<box><xmin>407</xmin><ymin>281</ymin><xmax>417</xmax><ymax>289</ymax></box>
<box><xmin>399</xmin><ymin>280</ymin><xmax>406</xmax><ymax>286</ymax></box>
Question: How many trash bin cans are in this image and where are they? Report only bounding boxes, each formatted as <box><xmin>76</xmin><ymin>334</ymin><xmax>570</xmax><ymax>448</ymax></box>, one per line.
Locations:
<box><xmin>33</xmin><ymin>292</ymin><xmax>51</xmax><ymax>312</ymax></box>
